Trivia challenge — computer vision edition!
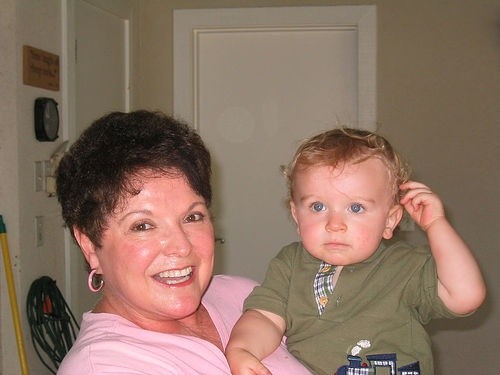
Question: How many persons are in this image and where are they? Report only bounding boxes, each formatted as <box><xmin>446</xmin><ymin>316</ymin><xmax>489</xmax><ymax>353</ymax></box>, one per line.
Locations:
<box><xmin>52</xmin><ymin>109</ymin><xmax>317</xmax><ymax>375</ymax></box>
<box><xmin>223</xmin><ymin>126</ymin><xmax>488</xmax><ymax>375</ymax></box>
